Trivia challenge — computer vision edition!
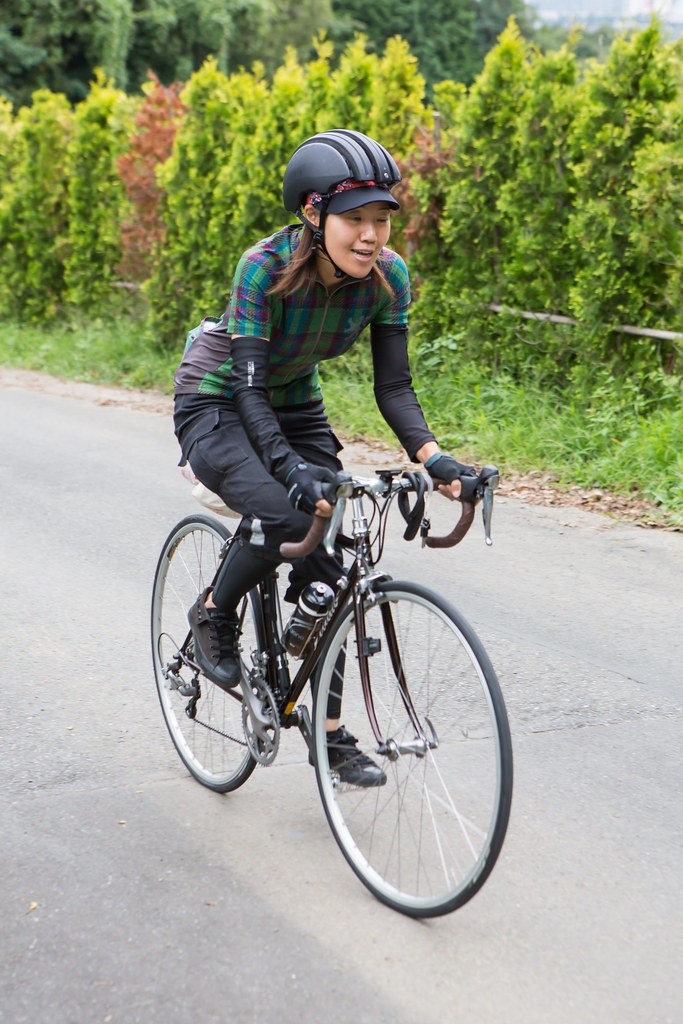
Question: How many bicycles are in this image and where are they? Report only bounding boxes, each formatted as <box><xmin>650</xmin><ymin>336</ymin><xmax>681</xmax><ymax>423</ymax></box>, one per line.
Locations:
<box><xmin>148</xmin><ymin>462</ymin><xmax>513</xmax><ymax>919</ymax></box>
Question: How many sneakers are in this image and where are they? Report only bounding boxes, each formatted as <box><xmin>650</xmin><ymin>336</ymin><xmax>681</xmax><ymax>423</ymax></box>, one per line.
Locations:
<box><xmin>188</xmin><ymin>587</ymin><xmax>242</xmax><ymax>687</ymax></box>
<box><xmin>309</xmin><ymin>724</ymin><xmax>388</xmax><ymax>787</ymax></box>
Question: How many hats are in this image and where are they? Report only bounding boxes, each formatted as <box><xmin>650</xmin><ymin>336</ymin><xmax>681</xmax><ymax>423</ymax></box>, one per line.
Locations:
<box><xmin>305</xmin><ymin>178</ymin><xmax>400</xmax><ymax>215</ymax></box>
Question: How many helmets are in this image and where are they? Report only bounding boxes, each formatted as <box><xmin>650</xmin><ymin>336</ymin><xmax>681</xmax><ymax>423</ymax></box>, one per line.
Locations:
<box><xmin>284</xmin><ymin>128</ymin><xmax>404</xmax><ymax>214</ymax></box>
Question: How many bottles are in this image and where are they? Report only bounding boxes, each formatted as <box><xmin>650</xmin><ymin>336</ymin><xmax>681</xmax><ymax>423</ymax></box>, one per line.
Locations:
<box><xmin>280</xmin><ymin>582</ymin><xmax>335</xmax><ymax>658</ymax></box>
<box><xmin>202</xmin><ymin>316</ymin><xmax>222</xmax><ymax>332</ymax></box>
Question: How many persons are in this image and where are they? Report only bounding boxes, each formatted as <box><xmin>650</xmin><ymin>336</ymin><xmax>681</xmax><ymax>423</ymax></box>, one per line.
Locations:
<box><xmin>173</xmin><ymin>129</ymin><xmax>476</xmax><ymax>785</ymax></box>
